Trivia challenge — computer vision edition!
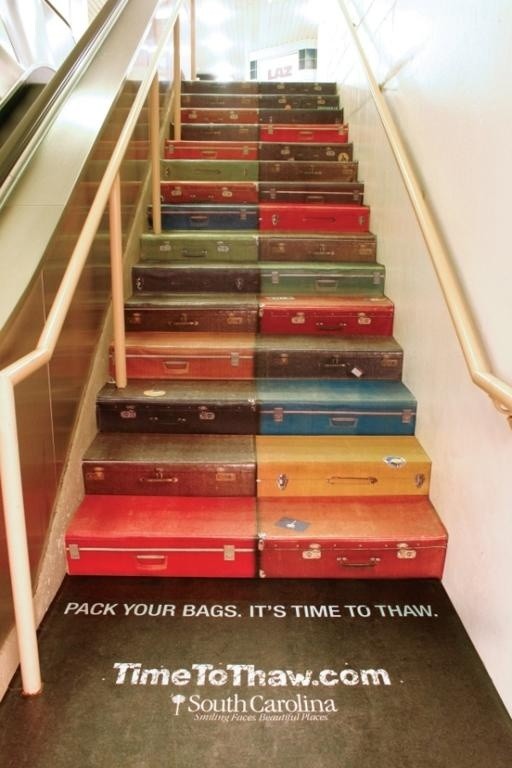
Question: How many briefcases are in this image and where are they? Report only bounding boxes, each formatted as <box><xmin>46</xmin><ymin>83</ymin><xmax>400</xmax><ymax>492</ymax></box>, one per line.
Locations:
<box><xmin>63</xmin><ymin>230</ymin><xmax>446</xmax><ymax>579</ymax></box>
